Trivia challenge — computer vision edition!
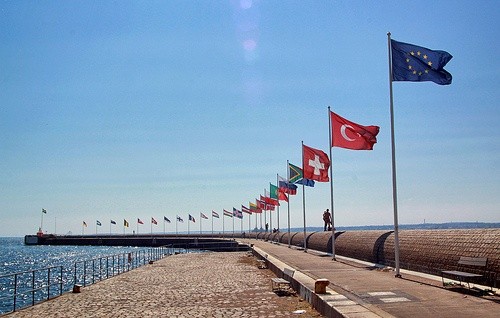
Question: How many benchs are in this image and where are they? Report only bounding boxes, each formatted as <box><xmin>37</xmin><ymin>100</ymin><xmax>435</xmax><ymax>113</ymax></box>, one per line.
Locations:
<box><xmin>256</xmin><ymin>253</ymin><xmax>268</xmax><ymax>269</ymax></box>
<box><xmin>441</xmin><ymin>256</ymin><xmax>489</xmax><ymax>294</ymax></box>
<box><xmin>270</xmin><ymin>267</ymin><xmax>296</xmax><ymax>294</ymax></box>
<box><xmin>246</xmin><ymin>248</ymin><xmax>255</xmax><ymax>257</ymax></box>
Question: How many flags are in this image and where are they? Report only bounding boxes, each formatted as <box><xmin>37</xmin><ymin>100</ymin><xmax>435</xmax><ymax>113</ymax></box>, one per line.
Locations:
<box><xmin>175</xmin><ymin>214</ymin><xmax>184</xmax><ymax>223</ymax></box>
<box><xmin>83</xmin><ymin>221</ymin><xmax>88</xmax><ymax>227</ymax></box>
<box><xmin>200</xmin><ymin>212</ymin><xmax>208</xmax><ymax>220</ymax></box>
<box><xmin>189</xmin><ymin>214</ymin><xmax>195</xmax><ymax>223</ymax></box>
<box><xmin>302</xmin><ymin>144</ymin><xmax>331</xmax><ymax>182</ymax></box>
<box><xmin>212</xmin><ymin>210</ymin><xmax>219</xmax><ymax>218</ymax></box>
<box><xmin>151</xmin><ymin>217</ymin><xmax>157</xmax><ymax>225</ymax></box>
<box><xmin>329</xmin><ymin>111</ymin><xmax>380</xmax><ymax>150</ymax></box>
<box><xmin>223</xmin><ymin>160</ymin><xmax>315</xmax><ymax>219</ymax></box>
<box><xmin>124</xmin><ymin>219</ymin><xmax>129</xmax><ymax>227</ymax></box>
<box><xmin>163</xmin><ymin>215</ymin><xmax>171</xmax><ymax>223</ymax></box>
<box><xmin>110</xmin><ymin>219</ymin><xmax>116</xmax><ymax>224</ymax></box>
<box><xmin>138</xmin><ymin>218</ymin><xmax>144</xmax><ymax>225</ymax></box>
<box><xmin>41</xmin><ymin>207</ymin><xmax>47</xmax><ymax>215</ymax></box>
<box><xmin>389</xmin><ymin>39</ymin><xmax>454</xmax><ymax>86</ymax></box>
<box><xmin>96</xmin><ymin>220</ymin><xmax>101</xmax><ymax>226</ymax></box>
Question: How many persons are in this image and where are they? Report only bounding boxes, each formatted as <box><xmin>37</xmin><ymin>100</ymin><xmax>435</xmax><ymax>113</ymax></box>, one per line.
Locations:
<box><xmin>322</xmin><ymin>210</ymin><xmax>332</xmax><ymax>232</ymax></box>
<box><xmin>273</xmin><ymin>228</ymin><xmax>276</xmax><ymax>232</ymax></box>
<box><xmin>265</xmin><ymin>223</ymin><xmax>269</xmax><ymax>232</ymax></box>
